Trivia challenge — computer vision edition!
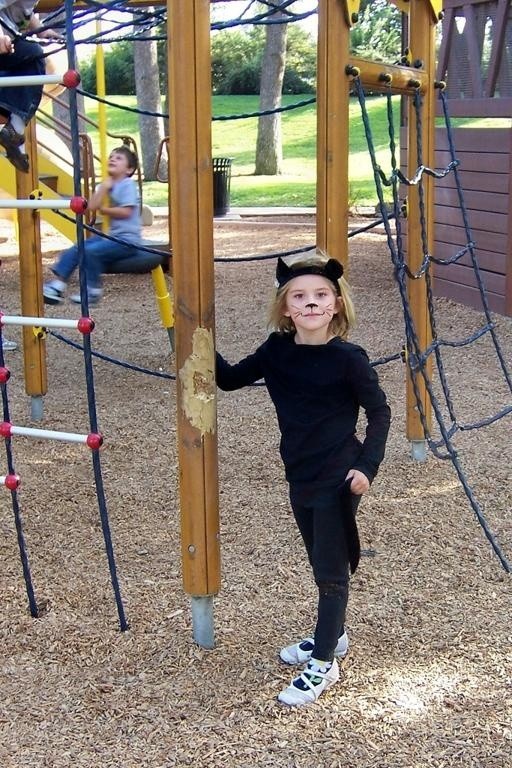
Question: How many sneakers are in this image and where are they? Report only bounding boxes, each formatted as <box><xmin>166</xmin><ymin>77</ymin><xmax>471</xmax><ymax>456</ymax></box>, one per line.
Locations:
<box><xmin>0</xmin><ymin>332</ymin><xmax>17</xmax><ymax>351</ymax></box>
<box><xmin>41</xmin><ymin>281</ymin><xmax>65</xmax><ymax>305</ymax></box>
<box><xmin>70</xmin><ymin>286</ymin><xmax>104</xmax><ymax>305</ymax></box>
<box><xmin>279</xmin><ymin>631</ymin><xmax>350</xmax><ymax>665</ymax></box>
<box><xmin>276</xmin><ymin>665</ymin><xmax>340</xmax><ymax>707</ymax></box>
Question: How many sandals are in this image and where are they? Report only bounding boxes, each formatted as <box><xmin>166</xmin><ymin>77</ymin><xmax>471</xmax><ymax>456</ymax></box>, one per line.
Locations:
<box><xmin>0</xmin><ymin>124</ymin><xmax>29</xmax><ymax>172</ymax></box>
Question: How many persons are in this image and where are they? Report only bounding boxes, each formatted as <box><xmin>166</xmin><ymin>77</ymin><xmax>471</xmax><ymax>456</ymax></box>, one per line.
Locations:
<box><xmin>216</xmin><ymin>248</ymin><xmax>390</xmax><ymax>707</ymax></box>
<box><xmin>42</xmin><ymin>146</ymin><xmax>141</xmax><ymax>306</ymax></box>
<box><xmin>0</xmin><ymin>0</ymin><xmax>64</xmax><ymax>172</ymax></box>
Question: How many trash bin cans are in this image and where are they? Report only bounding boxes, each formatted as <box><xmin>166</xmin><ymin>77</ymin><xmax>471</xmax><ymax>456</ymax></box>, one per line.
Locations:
<box><xmin>212</xmin><ymin>157</ymin><xmax>234</xmax><ymax>218</ymax></box>
